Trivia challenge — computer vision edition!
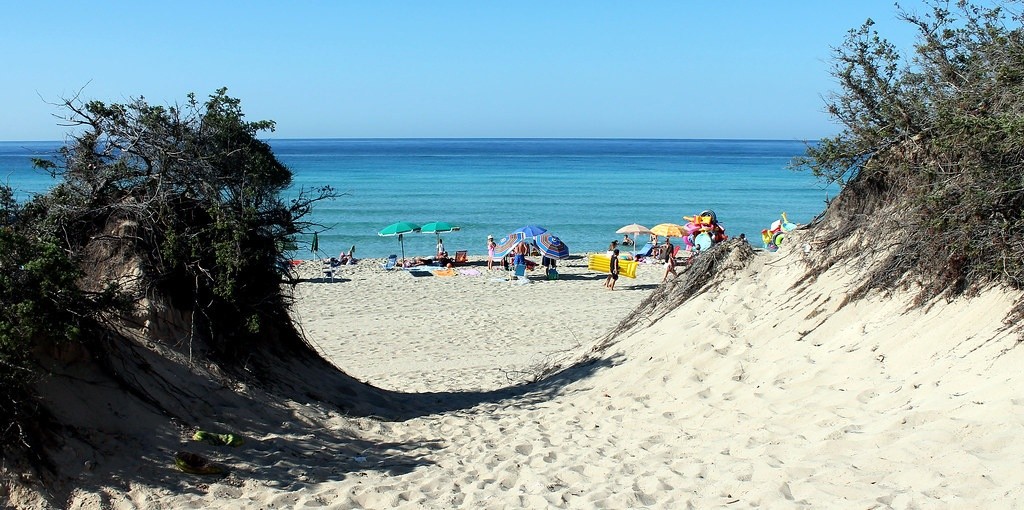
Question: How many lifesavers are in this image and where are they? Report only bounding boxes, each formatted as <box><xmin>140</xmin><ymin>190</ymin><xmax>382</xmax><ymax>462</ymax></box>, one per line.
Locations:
<box><xmin>683</xmin><ymin>209</ymin><xmax>728</xmax><ymax>251</ymax></box>
<box><xmin>762</xmin><ymin>210</ymin><xmax>796</xmax><ymax>253</ymax></box>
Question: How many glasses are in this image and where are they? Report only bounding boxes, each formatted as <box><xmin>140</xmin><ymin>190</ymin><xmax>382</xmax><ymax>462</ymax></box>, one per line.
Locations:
<box><xmin>665</xmin><ymin>241</ymin><xmax>667</xmax><ymax>242</ymax></box>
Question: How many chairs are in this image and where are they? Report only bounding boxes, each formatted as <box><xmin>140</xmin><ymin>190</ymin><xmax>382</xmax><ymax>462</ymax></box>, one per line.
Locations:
<box><xmin>319</xmin><ymin>258</ymin><xmax>333</xmax><ymax>282</ymax></box>
<box><xmin>511</xmin><ymin>264</ymin><xmax>527</xmax><ymax>279</ymax></box>
<box><xmin>632</xmin><ymin>242</ymin><xmax>655</xmax><ymax>255</ymax></box>
<box><xmin>453</xmin><ymin>250</ymin><xmax>468</xmax><ymax>263</ymax></box>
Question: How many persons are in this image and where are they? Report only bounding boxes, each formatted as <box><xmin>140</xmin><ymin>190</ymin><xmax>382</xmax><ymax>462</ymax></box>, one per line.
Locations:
<box><xmin>660</xmin><ymin>244</ymin><xmax>678</xmax><ymax>282</ymax></box>
<box><xmin>649</xmin><ymin>233</ymin><xmax>670</xmax><ymax>260</ymax></box>
<box><xmin>603</xmin><ymin>249</ymin><xmax>619</xmax><ymax>291</ymax></box>
<box><xmin>487</xmin><ymin>235</ymin><xmax>496</xmax><ymax>270</ymax></box>
<box><xmin>437</xmin><ymin>239</ymin><xmax>445</xmax><ymax>263</ymax></box>
<box><xmin>323</xmin><ymin>251</ymin><xmax>352</xmax><ymax>267</ymax></box>
<box><xmin>541</xmin><ymin>253</ymin><xmax>555</xmax><ymax>276</ymax></box>
<box><xmin>511</xmin><ymin>240</ymin><xmax>539</xmax><ymax>271</ymax></box>
<box><xmin>623</xmin><ymin>234</ymin><xmax>634</xmax><ymax>246</ymax></box>
<box><xmin>609</xmin><ymin>240</ymin><xmax>619</xmax><ymax>251</ymax></box>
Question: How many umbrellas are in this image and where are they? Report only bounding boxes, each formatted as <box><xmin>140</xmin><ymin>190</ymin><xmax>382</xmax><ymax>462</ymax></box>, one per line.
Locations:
<box><xmin>490</xmin><ymin>224</ymin><xmax>569</xmax><ymax>269</ymax></box>
<box><xmin>310</xmin><ymin>230</ymin><xmax>319</xmax><ymax>262</ymax></box>
<box><xmin>650</xmin><ymin>222</ymin><xmax>689</xmax><ymax>246</ymax></box>
<box><xmin>420</xmin><ymin>220</ymin><xmax>461</xmax><ymax>257</ymax></box>
<box><xmin>378</xmin><ymin>220</ymin><xmax>421</xmax><ymax>268</ymax></box>
<box><xmin>615</xmin><ymin>223</ymin><xmax>652</xmax><ymax>260</ymax></box>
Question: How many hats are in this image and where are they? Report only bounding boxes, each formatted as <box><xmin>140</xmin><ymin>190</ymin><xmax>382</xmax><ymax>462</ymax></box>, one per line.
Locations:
<box><xmin>488</xmin><ymin>234</ymin><xmax>493</xmax><ymax>239</ymax></box>
